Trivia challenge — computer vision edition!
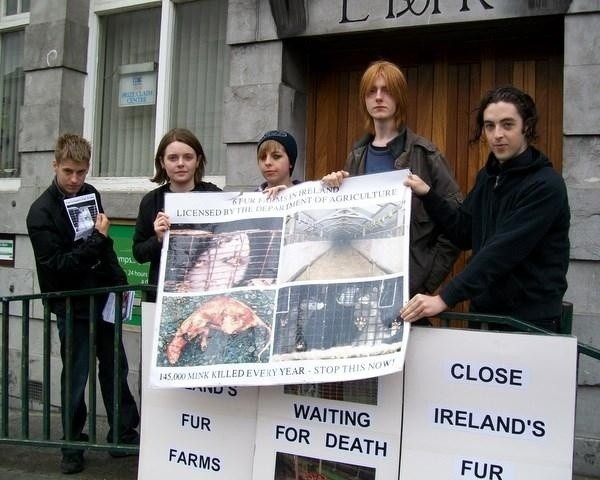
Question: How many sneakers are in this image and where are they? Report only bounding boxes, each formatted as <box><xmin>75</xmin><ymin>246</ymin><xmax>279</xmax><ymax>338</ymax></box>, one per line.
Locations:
<box><xmin>59</xmin><ymin>448</ymin><xmax>87</xmax><ymax>475</ymax></box>
<box><xmin>107</xmin><ymin>427</ymin><xmax>140</xmax><ymax>457</ymax></box>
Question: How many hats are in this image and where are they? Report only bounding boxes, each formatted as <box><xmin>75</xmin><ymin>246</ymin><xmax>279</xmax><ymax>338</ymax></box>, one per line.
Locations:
<box><xmin>256</xmin><ymin>130</ymin><xmax>298</xmax><ymax>177</ymax></box>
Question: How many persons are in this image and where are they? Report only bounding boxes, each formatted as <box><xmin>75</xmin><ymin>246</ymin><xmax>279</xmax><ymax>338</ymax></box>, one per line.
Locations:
<box><xmin>26</xmin><ymin>130</ymin><xmax>139</xmax><ymax>476</ymax></box>
<box><xmin>252</xmin><ymin>130</ymin><xmax>303</xmax><ymax>204</ymax></box>
<box><xmin>131</xmin><ymin>125</ymin><xmax>225</xmax><ymax>302</ymax></box>
<box><xmin>399</xmin><ymin>85</ymin><xmax>572</xmax><ymax>336</ymax></box>
<box><xmin>175</xmin><ymin>223</ymin><xmax>251</xmax><ymax>295</ymax></box>
<box><xmin>319</xmin><ymin>58</ymin><xmax>466</xmax><ymax>327</ymax></box>
